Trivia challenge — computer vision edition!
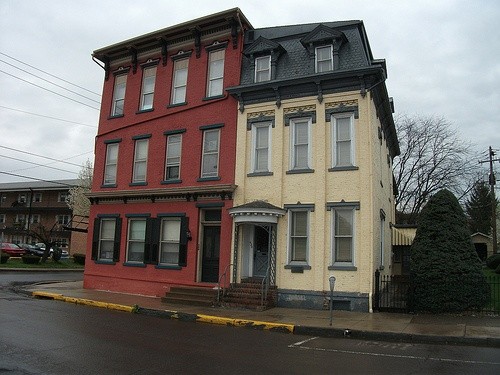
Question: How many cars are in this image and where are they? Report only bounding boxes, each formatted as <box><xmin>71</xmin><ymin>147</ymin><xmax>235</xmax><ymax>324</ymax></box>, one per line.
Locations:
<box><xmin>17</xmin><ymin>243</ymin><xmax>46</xmax><ymax>256</ymax></box>
<box><xmin>34</xmin><ymin>242</ymin><xmax>68</xmax><ymax>258</ymax></box>
<box><xmin>0</xmin><ymin>242</ymin><xmax>26</xmax><ymax>257</ymax></box>
